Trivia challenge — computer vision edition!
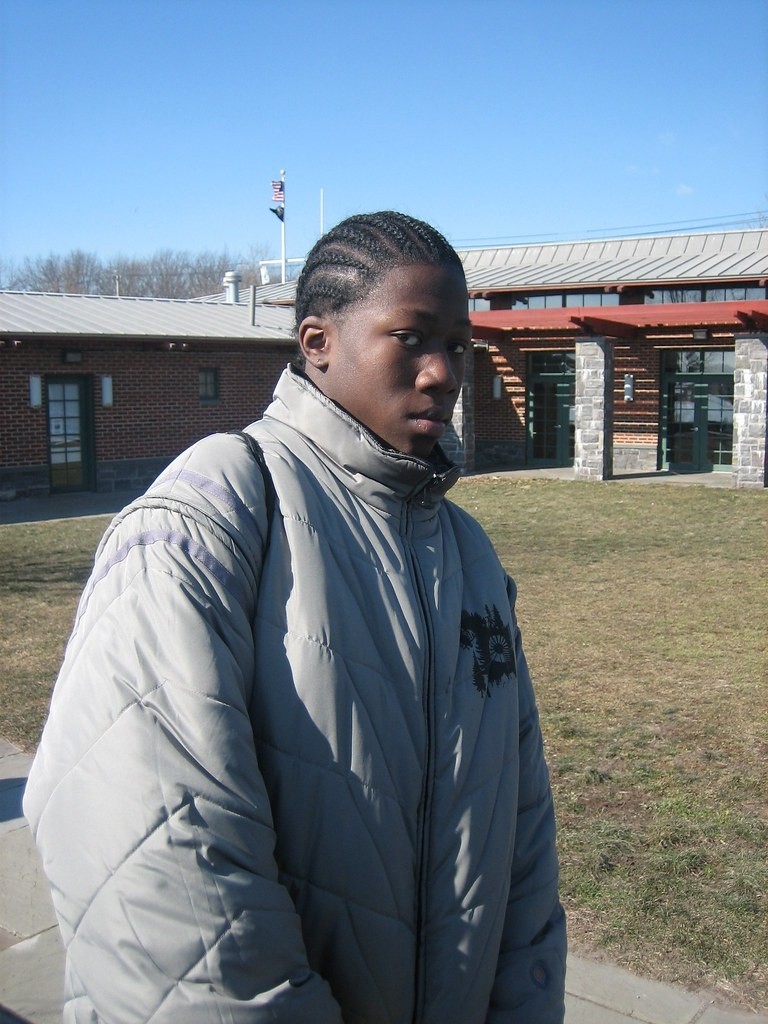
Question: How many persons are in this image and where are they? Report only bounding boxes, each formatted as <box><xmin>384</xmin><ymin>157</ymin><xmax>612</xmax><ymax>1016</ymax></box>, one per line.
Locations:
<box><xmin>27</xmin><ymin>212</ymin><xmax>570</xmax><ymax>1020</ymax></box>
